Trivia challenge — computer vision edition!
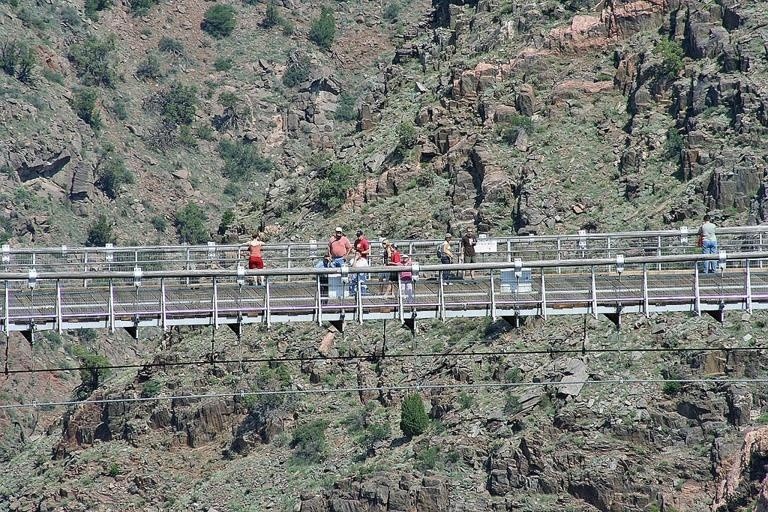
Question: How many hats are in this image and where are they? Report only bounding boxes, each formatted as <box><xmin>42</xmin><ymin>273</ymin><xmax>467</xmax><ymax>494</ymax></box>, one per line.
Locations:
<box><xmin>355</xmin><ymin>230</ymin><xmax>362</xmax><ymax>235</ymax></box>
<box><xmin>402</xmin><ymin>255</ymin><xmax>408</xmax><ymax>258</ymax></box>
<box><xmin>336</xmin><ymin>227</ymin><xmax>342</xmax><ymax>232</ymax></box>
<box><xmin>445</xmin><ymin>233</ymin><xmax>452</xmax><ymax>238</ymax></box>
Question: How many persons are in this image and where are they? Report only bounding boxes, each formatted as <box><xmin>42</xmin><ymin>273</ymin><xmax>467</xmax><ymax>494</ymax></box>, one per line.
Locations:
<box><xmin>316</xmin><ymin>227</ymin><xmax>372</xmax><ymax>299</ymax></box>
<box><xmin>377</xmin><ymin>240</ymin><xmax>414</xmax><ymax>304</ymax></box>
<box><xmin>460</xmin><ymin>227</ymin><xmax>477</xmax><ymax>280</ymax></box>
<box><xmin>440</xmin><ymin>233</ymin><xmax>456</xmax><ymax>285</ymax></box>
<box><xmin>698</xmin><ymin>214</ymin><xmax>718</xmax><ymax>273</ymax></box>
<box><xmin>246</xmin><ymin>233</ymin><xmax>266</xmax><ymax>286</ymax></box>
